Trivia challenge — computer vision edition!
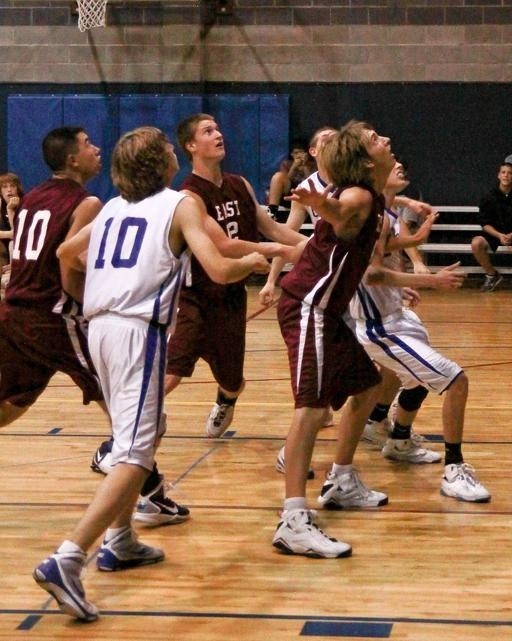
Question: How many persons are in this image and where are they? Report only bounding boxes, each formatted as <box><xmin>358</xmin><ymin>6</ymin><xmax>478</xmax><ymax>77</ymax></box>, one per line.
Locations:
<box><xmin>471</xmin><ymin>163</ymin><xmax>512</xmax><ymax>293</ymax></box>
<box><xmin>0</xmin><ymin>113</ymin><xmax>490</xmax><ymax>624</ymax></box>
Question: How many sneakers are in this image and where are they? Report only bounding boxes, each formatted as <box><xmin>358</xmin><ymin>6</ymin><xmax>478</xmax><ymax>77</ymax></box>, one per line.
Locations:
<box><xmin>206</xmin><ymin>392</ymin><xmax>240</xmax><ymax>443</ymax></box>
<box><xmin>97</xmin><ymin>530</ymin><xmax>166</xmax><ymax>572</ymax></box>
<box><xmin>317</xmin><ymin>471</ymin><xmax>389</xmax><ymax>511</ymax></box>
<box><xmin>442</xmin><ymin>463</ymin><xmax>492</xmax><ymax>504</ymax></box>
<box><xmin>135</xmin><ymin>483</ymin><xmax>191</xmax><ymax>525</ymax></box>
<box><xmin>271</xmin><ymin>509</ymin><xmax>352</xmax><ymax>559</ymax></box>
<box><xmin>480</xmin><ymin>272</ymin><xmax>503</xmax><ymax>293</ymax></box>
<box><xmin>361</xmin><ymin>415</ymin><xmax>442</xmax><ymax>464</ymax></box>
<box><xmin>277</xmin><ymin>445</ymin><xmax>314</xmax><ymax>479</ymax></box>
<box><xmin>91</xmin><ymin>444</ymin><xmax>171</xmax><ymax>487</ymax></box>
<box><xmin>32</xmin><ymin>552</ymin><xmax>99</xmax><ymax>621</ymax></box>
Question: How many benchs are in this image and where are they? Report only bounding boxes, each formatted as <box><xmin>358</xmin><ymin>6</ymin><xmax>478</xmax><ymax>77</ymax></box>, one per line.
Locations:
<box><xmin>256</xmin><ymin>204</ymin><xmax>511</xmax><ymax>274</ymax></box>
